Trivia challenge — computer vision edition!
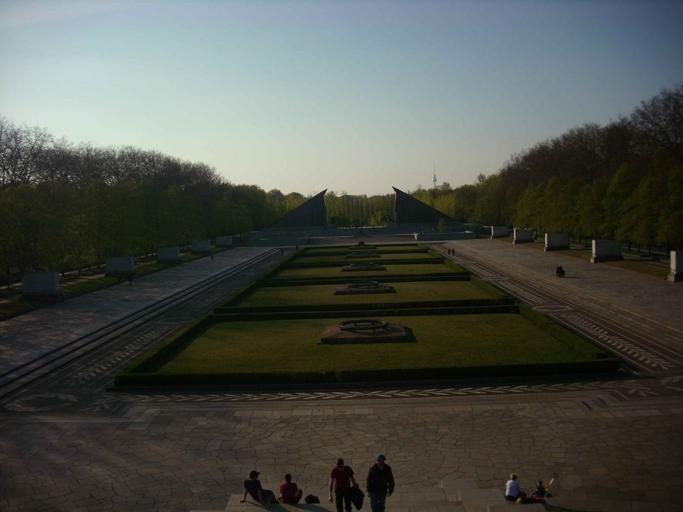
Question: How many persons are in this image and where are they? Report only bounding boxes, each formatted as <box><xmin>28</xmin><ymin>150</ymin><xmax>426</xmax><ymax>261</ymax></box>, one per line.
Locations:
<box><xmin>505</xmin><ymin>473</ymin><xmax>525</xmax><ymax>501</ymax></box>
<box><xmin>535</xmin><ymin>473</ymin><xmax>561</xmax><ymax>497</ymax></box>
<box><xmin>240</xmin><ymin>470</ymin><xmax>279</xmax><ymax>506</ymax></box>
<box><xmin>279</xmin><ymin>474</ymin><xmax>303</xmax><ymax>504</ymax></box>
<box><xmin>329</xmin><ymin>458</ymin><xmax>359</xmax><ymax>512</ymax></box>
<box><xmin>448</xmin><ymin>248</ymin><xmax>455</xmax><ymax>255</ymax></box>
<box><xmin>367</xmin><ymin>455</ymin><xmax>395</xmax><ymax>512</ymax></box>
